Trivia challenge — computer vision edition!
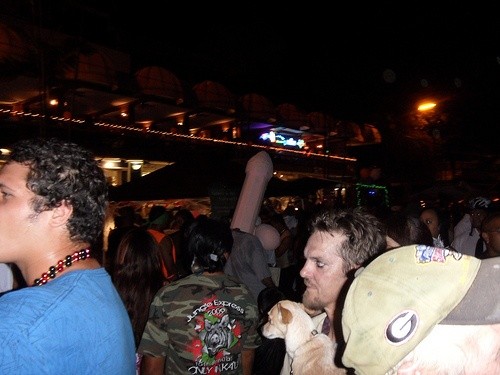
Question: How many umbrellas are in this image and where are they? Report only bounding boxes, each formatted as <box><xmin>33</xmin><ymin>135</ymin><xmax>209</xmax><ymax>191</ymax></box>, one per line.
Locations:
<box><xmin>104</xmin><ymin>143</ymin><xmax>340</xmax><ymax>213</ymax></box>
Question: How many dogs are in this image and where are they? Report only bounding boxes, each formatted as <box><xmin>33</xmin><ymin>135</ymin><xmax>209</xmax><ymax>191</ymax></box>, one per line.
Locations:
<box><xmin>260</xmin><ymin>299</ymin><xmax>355</xmax><ymax>375</ymax></box>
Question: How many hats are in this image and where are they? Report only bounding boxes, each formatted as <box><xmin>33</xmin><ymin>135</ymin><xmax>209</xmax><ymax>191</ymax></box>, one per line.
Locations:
<box><xmin>468</xmin><ymin>196</ymin><xmax>492</xmax><ymax>210</ymax></box>
<box><xmin>341</xmin><ymin>244</ymin><xmax>500</xmax><ymax>375</ymax></box>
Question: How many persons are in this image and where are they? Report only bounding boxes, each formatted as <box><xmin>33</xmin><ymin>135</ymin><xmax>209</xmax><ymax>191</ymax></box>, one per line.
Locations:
<box><xmin>90</xmin><ymin>195</ymin><xmax>500</xmax><ymax>375</ymax></box>
<box><xmin>0</xmin><ymin>137</ymin><xmax>135</xmax><ymax>375</ymax></box>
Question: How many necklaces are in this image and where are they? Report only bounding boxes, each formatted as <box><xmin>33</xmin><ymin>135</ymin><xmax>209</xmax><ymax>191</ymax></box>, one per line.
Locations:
<box><xmin>26</xmin><ymin>247</ymin><xmax>93</xmax><ymax>287</ymax></box>
